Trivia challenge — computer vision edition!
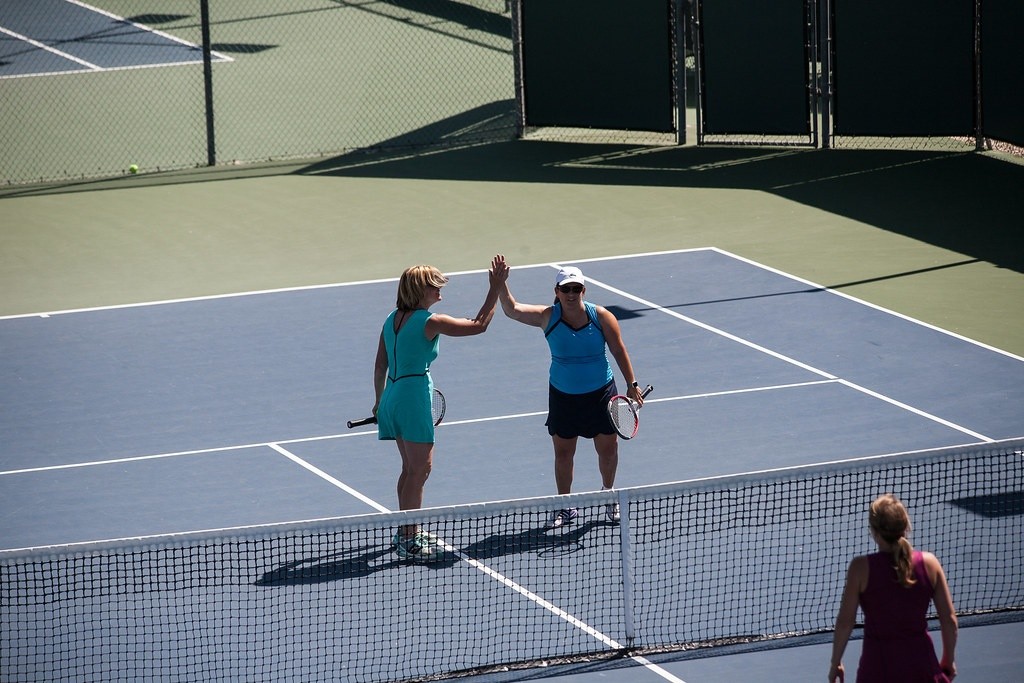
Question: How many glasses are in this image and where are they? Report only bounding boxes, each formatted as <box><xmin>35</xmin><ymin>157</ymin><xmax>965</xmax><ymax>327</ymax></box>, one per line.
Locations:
<box><xmin>556</xmin><ymin>285</ymin><xmax>584</xmax><ymax>294</ymax></box>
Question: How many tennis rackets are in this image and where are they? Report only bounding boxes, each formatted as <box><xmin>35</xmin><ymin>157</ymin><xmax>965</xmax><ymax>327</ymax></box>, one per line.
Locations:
<box><xmin>347</xmin><ymin>386</ymin><xmax>446</xmax><ymax>428</ymax></box>
<box><xmin>609</xmin><ymin>385</ymin><xmax>653</xmax><ymax>440</ymax></box>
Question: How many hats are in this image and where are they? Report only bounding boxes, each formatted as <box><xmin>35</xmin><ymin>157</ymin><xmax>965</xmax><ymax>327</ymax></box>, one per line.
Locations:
<box><xmin>556</xmin><ymin>267</ymin><xmax>584</xmax><ymax>286</ymax></box>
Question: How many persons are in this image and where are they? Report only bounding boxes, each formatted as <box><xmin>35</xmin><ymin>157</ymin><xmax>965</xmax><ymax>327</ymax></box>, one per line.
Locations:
<box><xmin>369</xmin><ymin>259</ymin><xmax>508</xmax><ymax>562</ymax></box>
<box><xmin>491</xmin><ymin>254</ymin><xmax>645</xmax><ymax>529</ymax></box>
<box><xmin>825</xmin><ymin>493</ymin><xmax>959</xmax><ymax>683</ymax></box>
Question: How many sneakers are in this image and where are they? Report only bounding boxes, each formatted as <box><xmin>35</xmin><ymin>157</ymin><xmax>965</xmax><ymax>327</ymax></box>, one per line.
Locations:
<box><xmin>392</xmin><ymin>530</ymin><xmax>440</xmax><ymax>545</ymax></box>
<box><xmin>395</xmin><ymin>539</ymin><xmax>446</xmax><ymax>563</ymax></box>
<box><xmin>543</xmin><ymin>508</ymin><xmax>580</xmax><ymax>529</ymax></box>
<box><xmin>605</xmin><ymin>503</ymin><xmax>621</xmax><ymax>522</ymax></box>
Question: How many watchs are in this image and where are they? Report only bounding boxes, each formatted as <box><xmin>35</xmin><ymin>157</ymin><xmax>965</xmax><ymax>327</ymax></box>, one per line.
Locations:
<box><xmin>627</xmin><ymin>381</ymin><xmax>638</xmax><ymax>389</ymax></box>
